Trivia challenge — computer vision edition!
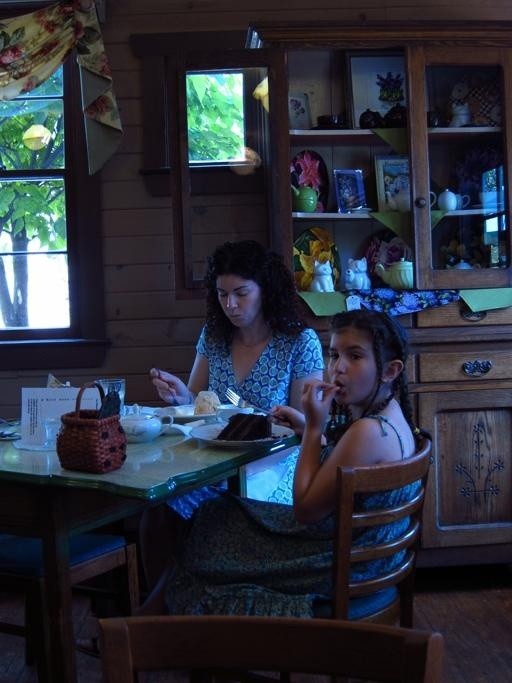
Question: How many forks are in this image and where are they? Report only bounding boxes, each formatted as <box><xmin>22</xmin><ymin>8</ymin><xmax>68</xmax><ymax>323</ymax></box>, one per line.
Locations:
<box><xmin>223</xmin><ymin>387</ymin><xmax>274</xmax><ymax>416</ymax></box>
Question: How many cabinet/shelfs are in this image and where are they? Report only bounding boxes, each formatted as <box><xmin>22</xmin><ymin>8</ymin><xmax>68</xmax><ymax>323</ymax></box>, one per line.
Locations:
<box><xmin>320</xmin><ymin>332</ymin><xmax>512</xmax><ymax>569</ymax></box>
<box><xmin>253</xmin><ymin>19</ymin><xmax>512</xmax><ymax>331</ymax></box>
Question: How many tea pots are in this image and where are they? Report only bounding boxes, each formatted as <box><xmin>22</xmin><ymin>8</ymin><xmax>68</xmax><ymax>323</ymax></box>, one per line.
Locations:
<box><xmin>292</xmin><ymin>183</ymin><xmax>319</xmax><ymax>213</ymax></box>
<box><xmin>385</xmin><ymin>183</ymin><xmax>436</xmax><ymax>213</ymax></box>
<box><xmin>119</xmin><ymin>402</ymin><xmax>175</xmax><ymax>444</ymax></box>
<box><xmin>375</xmin><ymin>257</ymin><xmax>415</xmax><ymax>290</ymax></box>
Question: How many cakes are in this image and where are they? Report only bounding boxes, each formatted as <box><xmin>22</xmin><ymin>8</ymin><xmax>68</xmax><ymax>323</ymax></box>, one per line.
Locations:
<box><xmin>192</xmin><ymin>390</ymin><xmax>218</xmax><ymax>414</ymax></box>
<box><xmin>214</xmin><ymin>412</ymin><xmax>275</xmax><ymax>442</ymax></box>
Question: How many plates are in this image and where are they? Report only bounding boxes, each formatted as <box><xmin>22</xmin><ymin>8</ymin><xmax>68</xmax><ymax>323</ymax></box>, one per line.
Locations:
<box><xmin>152</xmin><ymin>403</ymin><xmax>257</xmax><ymax>421</ymax></box>
<box><xmin>189</xmin><ymin>423</ymin><xmax>296</xmax><ymax>449</ymax></box>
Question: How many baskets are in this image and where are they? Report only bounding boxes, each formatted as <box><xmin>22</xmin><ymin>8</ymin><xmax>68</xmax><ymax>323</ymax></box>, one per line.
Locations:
<box><xmin>56</xmin><ymin>382</ymin><xmax>128</xmax><ymax>474</ymax></box>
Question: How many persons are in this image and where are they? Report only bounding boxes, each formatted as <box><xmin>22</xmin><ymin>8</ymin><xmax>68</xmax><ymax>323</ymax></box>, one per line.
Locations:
<box><xmin>71</xmin><ymin>308</ymin><xmax>432</xmax><ymax>660</ymax></box>
<box><xmin>146</xmin><ymin>236</ymin><xmax>327</xmax><ymax>562</ymax></box>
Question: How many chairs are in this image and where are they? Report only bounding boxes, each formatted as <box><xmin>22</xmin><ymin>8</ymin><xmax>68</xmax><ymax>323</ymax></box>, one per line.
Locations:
<box><xmin>0</xmin><ymin>483</ymin><xmax>141</xmax><ymax>683</ymax></box>
<box><xmin>335</xmin><ymin>427</ymin><xmax>432</xmax><ymax>628</ymax></box>
<box><xmin>95</xmin><ymin>616</ymin><xmax>444</xmax><ymax>681</ymax></box>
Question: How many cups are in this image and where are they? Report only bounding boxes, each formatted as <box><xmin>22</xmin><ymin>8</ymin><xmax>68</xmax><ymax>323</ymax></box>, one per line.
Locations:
<box><xmin>94</xmin><ymin>377</ymin><xmax>127</xmax><ymax>413</ymax></box>
<box><xmin>455</xmin><ymin>193</ymin><xmax>472</xmax><ymax>211</ymax></box>
<box><xmin>215</xmin><ymin>404</ymin><xmax>255</xmax><ymax>423</ymax></box>
<box><xmin>437</xmin><ymin>188</ymin><xmax>457</xmax><ymax>211</ymax></box>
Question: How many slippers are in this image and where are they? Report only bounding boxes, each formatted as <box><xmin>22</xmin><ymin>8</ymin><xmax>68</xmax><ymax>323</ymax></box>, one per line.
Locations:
<box><xmin>73</xmin><ymin>635</ymin><xmax>102</xmax><ymax>660</ymax></box>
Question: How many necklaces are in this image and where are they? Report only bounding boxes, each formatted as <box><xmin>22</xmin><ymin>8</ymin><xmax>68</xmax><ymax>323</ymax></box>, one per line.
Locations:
<box><xmin>238</xmin><ymin>326</ymin><xmax>272</xmax><ymax>348</ymax></box>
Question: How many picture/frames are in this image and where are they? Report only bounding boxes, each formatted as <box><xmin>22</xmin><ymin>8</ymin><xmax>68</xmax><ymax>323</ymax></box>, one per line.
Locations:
<box><xmin>334</xmin><ymin>170</ymin><xmax>367</xmax><ymax>214</ymax></box>
<box><xmin>375</xmin><ymin>155</ymin><xmax>411</xmax><ymax>214</ymax></box>
<box><xmin>344</xmin><ymin>50</ymin><xmax>410</xmax><ymax>129</ymax></box>
